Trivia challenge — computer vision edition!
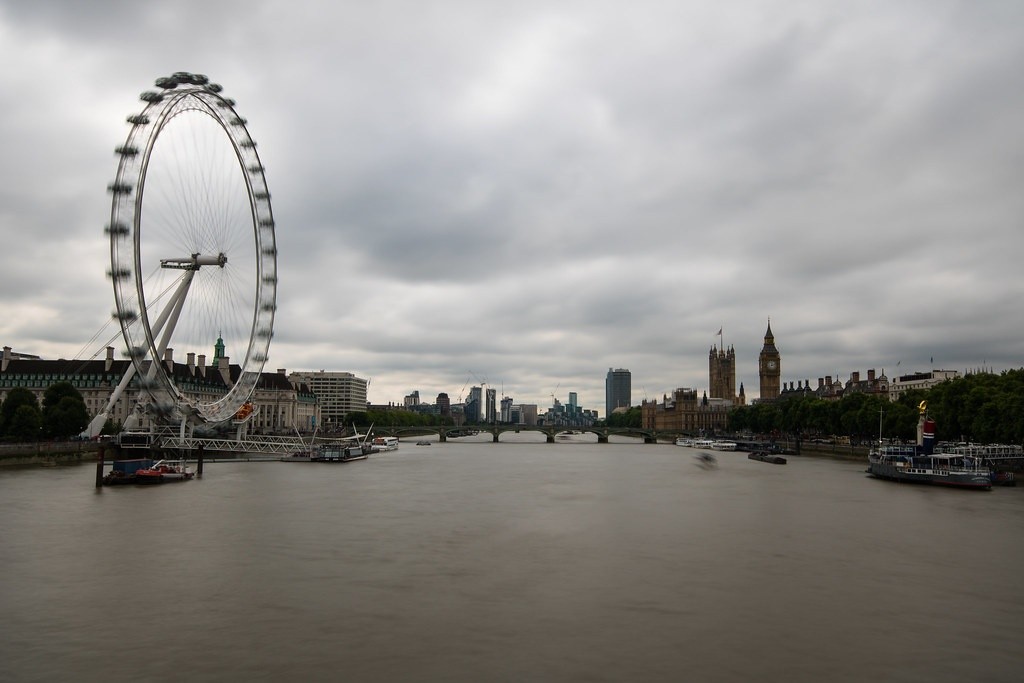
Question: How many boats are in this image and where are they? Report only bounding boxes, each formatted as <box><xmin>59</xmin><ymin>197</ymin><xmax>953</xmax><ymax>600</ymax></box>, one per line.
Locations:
<box><xmin>865</xmin><ymin>406</ymin><xmax>993</xmax><ymax>490</ymax></box>
<box><xmin>309</xmin><ymin>441</ymin><xmax>370</xmax><ymax>463</ymax></box>
<box><xmin>711</xmin><ymin>440</ymin><xmax>738</xmax><ymax>453</ymax></box>
<box><xmin>136</xmin><ymin>457</ymin><xmax>197</xmax><ymax>485</ymax></box>
<box><xmin>693</xmin><ymin>438</ymin><xmax>713</xmax><ymax>449</ymax></box>
<box><xmin>675</xmin><ymin>437</ymin><xmax>695</xmax><ymax>447</ymax></box>
<box><xmin>369</xmin><ymin>437</ymin><xmax>400</xmax><ymax>453</ymax></box>
